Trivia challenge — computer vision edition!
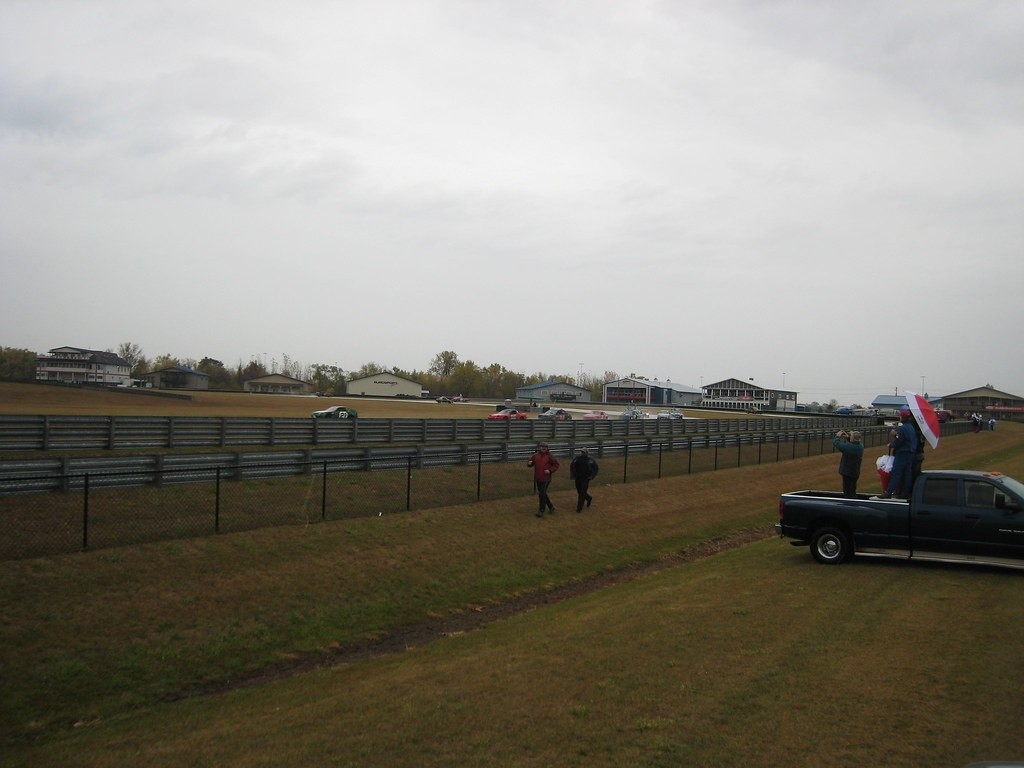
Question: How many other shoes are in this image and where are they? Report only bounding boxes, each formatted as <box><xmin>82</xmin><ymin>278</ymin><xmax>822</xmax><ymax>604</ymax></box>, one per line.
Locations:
<box><xmin>877</xmin><ymin>492</ymin><xmax>892</xmax><ymax>499</ymax></box>
<box><xmin>536</xmin><ymin>511</ymin><xmax>543</xmax><ymax>517</ymax></box>
<box><xmin>548</xmin><ymin>507</ymin><xmax>555</xmax><ymax>513</ymax></box>
<box><xmin>587</xmin><ymin>497</ymin><xmax>592</xmax><ymax>507</ymax></box>
<box><xmin>896</xmin><ymin>494</ymin><xmax>909</xmax><ymax>499</ymax></box>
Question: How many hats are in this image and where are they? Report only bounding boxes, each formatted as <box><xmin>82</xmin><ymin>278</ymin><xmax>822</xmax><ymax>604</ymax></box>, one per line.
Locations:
<box><xmin>539</xmin><ymin>442</ymin><xmax>548</xmax><ymax>447</ymax></box>
<box><xmin>580</xmin><ymin>447</ymin><xmax>588</xmax><ymax>453</ymax></box>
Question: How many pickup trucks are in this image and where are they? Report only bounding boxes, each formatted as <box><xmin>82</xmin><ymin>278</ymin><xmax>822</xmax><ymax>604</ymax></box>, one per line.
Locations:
<box><xmin>775</xmin><ymin>469</ymin><xmax>1024</xmax><ymax>572</ymax></box>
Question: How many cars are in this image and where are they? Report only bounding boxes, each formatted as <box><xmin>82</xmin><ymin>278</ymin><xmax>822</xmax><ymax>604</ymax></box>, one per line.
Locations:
<box><xmin>488</xmin><ymin>409</ymin><xmax>529</xmax><ymax>420</ymax></box>
<box><xmin>657</xmin><ymin>410</ymin><xmax>671</xmax><ymax>419</ymax></box>
<box><xmin>585</xmin><ymin>411</ymin><xmax>609</xmax><ymax>419</ymax></box>
<box><xmin>436</xmin><ymin>396</ymin><xmax>453</xmax><ymax>404</ymax></box>
<box><xmin>452</xmin><ymin>396</ymin><xmax>468</xmax><ymax>402</ymax></box>
<box><xmin>311</xmin><ymin>406</ymin><xmax>358</xmax><ymax>420</ymax></box>
<box><xmin>833</xmin><ymin>406</ymin><xmax>852</xmax><ymax>414</ymax></box>
<box><xmin>746</xmin><ymin>406</ymin><xmax>761</xmax><ymax>414</ymax></box>
<box><xmin>538</xmin><ymin>409</ymin><xmax>572</xmax><ymax>420</ymax></box>
<box><xmin>669</xmin><ymin>409</ymin><xmax>684</xmax><ymax>419</ymax></box>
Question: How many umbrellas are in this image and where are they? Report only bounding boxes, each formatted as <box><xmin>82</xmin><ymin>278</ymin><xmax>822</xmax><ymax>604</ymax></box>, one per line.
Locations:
<box><xmin>904</xmin><ymin>391</ymin><xmax>940</xmax><ymax>450</ymax></box>
<box><xmin>876</xmin><ymin>446</ymin><xmax>895</xmax><ymax>494</ymax></box>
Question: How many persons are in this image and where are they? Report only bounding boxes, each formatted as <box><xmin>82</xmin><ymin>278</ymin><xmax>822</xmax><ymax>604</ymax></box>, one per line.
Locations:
<box><xmin>877</xmin><ymin>410</ymin><xmax>925</xmax><ymax>499</ymax></box>
<box><xmin>988</xmin><ymin>417</ymin><xmax>996</xmax><ymax>432</ymax></box>
<box><xmin>570</xmin><ymin>447</ymin><xmax>598</xmax><ymax>513</ymax></box>
<box><xmin>833</xmin><ymin>430</ymin><xmax>864</xmax><ymax>499</ymax></box>
<box><xmin>527</xmin><ymin>442</ymin><xmax>560</xmax><ymax>517</ymax></box>
<box><xmin>972</xmin><ymin>412</ymin><xmax>983</xmax><ymax>433</ymax></box>
